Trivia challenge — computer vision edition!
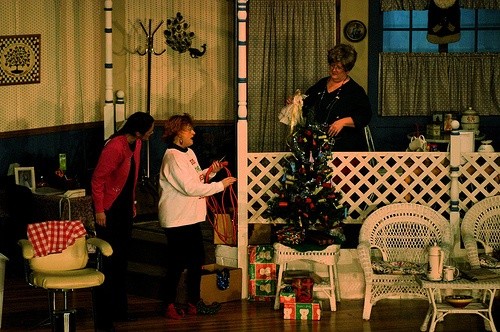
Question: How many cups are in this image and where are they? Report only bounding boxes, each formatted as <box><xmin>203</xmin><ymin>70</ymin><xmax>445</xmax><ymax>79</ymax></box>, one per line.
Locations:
<box><xmin>409</xmin><ymin>135</ymin><xmax>427</xmax><ymax>152</ymax></box>
<box><xmin>442</xmin><ymin>265</ymin><xmax>460</xmax><ymax>282</ymax></box>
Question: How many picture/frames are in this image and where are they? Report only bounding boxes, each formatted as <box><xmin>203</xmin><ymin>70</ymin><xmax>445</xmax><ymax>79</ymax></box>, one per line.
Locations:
<box><xmin>13</xmin><ymin>166</ymin><xmax>35</xmax><ymax>191</ymax></box>
<box><xmin>344</xmin><ymin>19</ymin><xmax>367</xmax><ymax>42</ymax></box>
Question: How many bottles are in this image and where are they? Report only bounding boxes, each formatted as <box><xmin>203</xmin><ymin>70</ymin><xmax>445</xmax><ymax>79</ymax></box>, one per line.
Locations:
<box><xmin>478</xmin><ymin>140</ymin><xmax>494</xmax><ymax>153</ymax></box>
<box><xmin>461</xmin><ymin>107</ymin><xmax>480</xmax><ymax>130</ymax></box>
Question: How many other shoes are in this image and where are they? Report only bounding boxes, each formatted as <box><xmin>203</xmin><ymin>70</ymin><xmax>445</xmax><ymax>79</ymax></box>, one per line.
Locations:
<box><xmin>164</xmin><ymin>304</ymin><xmax>182</xmax><ymax>320</ymax></box>
<box><xmin>187</xmin><ymin>299</ymin><xmax>219</xmax><ymax>315</ymax></box>
<box><xmin>117</xmin><ymin>314</ymin><xmax>137</xmax><ymax>322</ymax></box>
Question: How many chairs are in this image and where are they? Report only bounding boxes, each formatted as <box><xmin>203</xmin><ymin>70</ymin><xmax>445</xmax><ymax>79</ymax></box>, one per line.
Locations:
<box><xmin>462</xmin><ymin>195</ymin><xmax>500</xmax><ymax>331</ymax></box>
<box><xmin>362</xmin><ymin>126</ymin><xmax>405</xmax><ymax>211</ymax></box>
<box><xmin>18</xmin><ymin>220</ymin><xmax>113</xmax><ymax>331</ymax></box>
<box><xmin>355</xmin><ymin>203</ymin><xmax>453</xmax><ymax>320</ymax></box>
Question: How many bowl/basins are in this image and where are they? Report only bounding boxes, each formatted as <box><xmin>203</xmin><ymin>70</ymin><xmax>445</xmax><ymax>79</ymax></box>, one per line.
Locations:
<box><xmin>444</xmin><ymin>295</ymin><xmax>474</xmax><ymax>308</ymax></box>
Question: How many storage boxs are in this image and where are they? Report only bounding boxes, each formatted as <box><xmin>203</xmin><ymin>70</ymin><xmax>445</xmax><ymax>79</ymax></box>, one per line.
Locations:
<box><xmin>174</xmin><ymin>212</ymin><xmax>242</xmax><ymax>305</ymax></box>
<box><xmin>248</xmin><ymin>245</ymin><xmax>321</xmax><ymax>320</ymax></box>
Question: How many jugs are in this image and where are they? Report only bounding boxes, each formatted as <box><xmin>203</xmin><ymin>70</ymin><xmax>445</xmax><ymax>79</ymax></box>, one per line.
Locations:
<box><xmin>427</xmin><ymin>243</ymin><xmax>444</xmax><ymax>281</ymax></box>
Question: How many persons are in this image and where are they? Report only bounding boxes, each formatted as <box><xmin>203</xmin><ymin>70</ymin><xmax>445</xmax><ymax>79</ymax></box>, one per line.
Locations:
<box><xmin>91</xmin><ymin>112</ymin><xmax>154</xmax><ymax>332</ymax></box>
<box><xmin>154</xmin><ymin>113</ymin><xmax>236</xmax><ymax>319</ymax></box>
<box><xmin>285</xmin><ymin>43</ymin><xmax>373</xmax><ymax>191</ymax></box>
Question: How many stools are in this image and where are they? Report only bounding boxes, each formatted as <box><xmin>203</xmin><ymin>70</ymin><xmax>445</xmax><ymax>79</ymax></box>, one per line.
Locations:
<box><xmin>271</xmin><ymin>242</ymin><xmax>341</xmax><ymax>312</ymax></box>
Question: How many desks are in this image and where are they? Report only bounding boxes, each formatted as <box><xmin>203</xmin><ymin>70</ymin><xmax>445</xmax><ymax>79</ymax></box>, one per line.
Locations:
<box><xmin>415</xmin><ymin>274</ymin><xmax>500</xmax><ymax>332</ymax></box>
<box><xmin>408</xmin><ymin>129</ymin><xmax>486</xmax><ymax>154</ymax></box>
<box><xmin>11</xmin><ymin>185</ymin><xmax>93</xmax><ymax>233</ymax></box>
